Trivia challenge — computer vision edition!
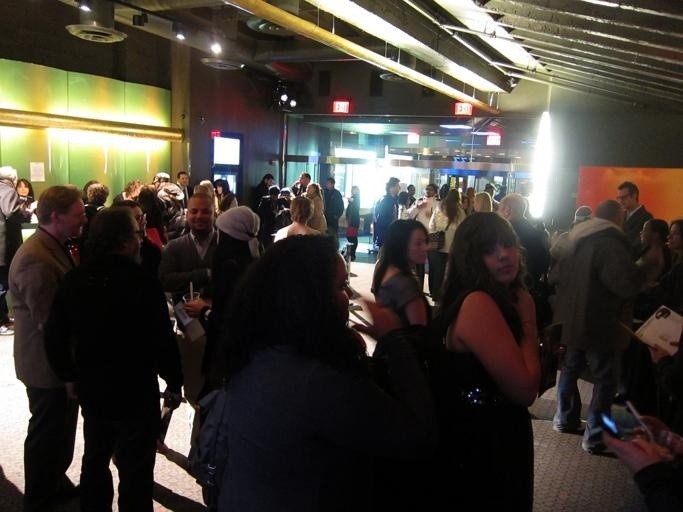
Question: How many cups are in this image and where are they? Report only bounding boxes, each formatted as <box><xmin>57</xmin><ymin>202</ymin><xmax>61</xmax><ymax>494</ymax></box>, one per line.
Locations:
<box><xmin>181</xmin><ymin>291</ymin><xmax>199</xmax><ymax>318</ymax></box>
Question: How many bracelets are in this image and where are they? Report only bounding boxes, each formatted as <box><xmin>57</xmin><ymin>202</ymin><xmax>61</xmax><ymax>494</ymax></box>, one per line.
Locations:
<box><xmin>521</xmin><ymin>320</ymin><xmax>536</xmax><ymax>327</ymax></box>
<box><xmin>519</xmin><ymin>333</ymin><xmax>537</xmax><ymax>337</ymax></box>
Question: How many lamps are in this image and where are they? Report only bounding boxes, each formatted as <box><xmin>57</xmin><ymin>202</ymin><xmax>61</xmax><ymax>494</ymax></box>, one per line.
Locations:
<box><xmin>484</xmin><ymin>134</ymin><xmax>502</xmax><ymax>147</ymax></box>
<box><xmin>113</xmin><ymin>0</ymin><xmax>239</xmax><ymax>56</ymax></box>
<box><xmin>265</xmin><ymin>77</ymin><xmax>300</xmax><ymax>118</ymax></box>
<box><xmin>330</xmin><ymin>98</ymin><xmax>350</xmax><ymax>115</ymax></box>
<box><xmin>452</xmin><ymin>100</ymin><xmax>473</xmax><ymax>118</ymax></box>
<box><xmin>507</xmin><ymin>72</ymin><xmax>519</xmax><ymax>89</ymax></box>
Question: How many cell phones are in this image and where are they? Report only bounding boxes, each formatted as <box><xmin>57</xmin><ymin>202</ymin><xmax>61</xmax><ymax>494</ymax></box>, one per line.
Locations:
<box><xmin>593</xmin><ymin>408</ymin><xmax>634</xmax><ymax>442</ymax></box>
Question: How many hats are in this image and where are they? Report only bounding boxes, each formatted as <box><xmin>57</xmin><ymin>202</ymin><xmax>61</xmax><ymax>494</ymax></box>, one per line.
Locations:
<box><xmin>215</xmin><ymin>205</ymin><xmax>260</xmax><ymax>241</ymax></box>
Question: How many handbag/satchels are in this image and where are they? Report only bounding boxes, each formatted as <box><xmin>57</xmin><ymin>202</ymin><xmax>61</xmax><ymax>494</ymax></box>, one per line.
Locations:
<box><xmin>429</xmin><ymin>231</ymin><xmax>445</xmax><ymax>251</ymax></box>
<box><xmin>189</xmin><ymin>387</ymin><xmax>225</xmax><ymax>488</ymax></box>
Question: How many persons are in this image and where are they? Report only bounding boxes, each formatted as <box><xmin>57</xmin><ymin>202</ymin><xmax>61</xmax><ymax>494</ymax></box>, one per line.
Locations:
<box><xmin>374</xmin><ymin>178</ymin><xmax>683</xmax><ymax>453</ymax></box>
<box><xmin>606</xmin><ymin>401</ymin><xmax>682</xmax><ymax>511</ymax></box>
<box><xmin>434</xmin><ymin>212</ymin><xmax>542</xmax><ymax>511</ymax></box>
<box><xmin>0</xmin><ymin>167</ymin><xmax>35</xmax><ymax>335</ymax></box>
<box><xmin>84</xmin><ymin>174</ymin><xmax>359</xmax><ymax>329</ymax></box>
<box><xmin>9</xmin><ymin>186</ymin><xmax>88</xmax><ymax>511</ymax></box>
<box><xmin>42</xmin><ymin>205</ymin><xmax>183</xmax><ymax>511</ymax></box>
<box><xmin>183</xmin><ymin>235</ymin><xmax>404</xmax><ymax>511</ymax></box>
